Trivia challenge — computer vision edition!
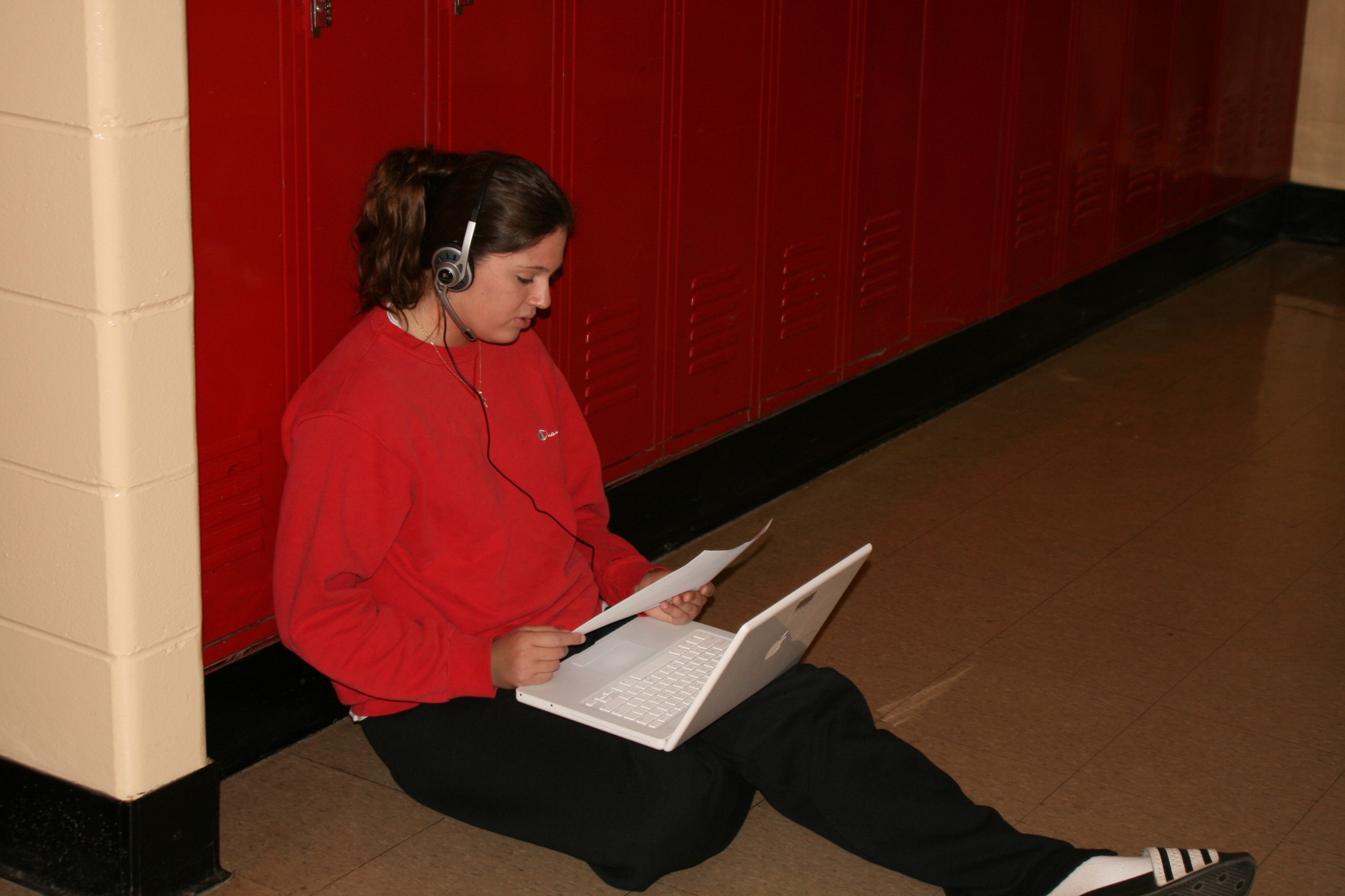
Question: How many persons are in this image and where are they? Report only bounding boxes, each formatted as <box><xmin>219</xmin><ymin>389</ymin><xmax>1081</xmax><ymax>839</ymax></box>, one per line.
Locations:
<box><xmin>274</xmin><ymin>143</ymin><xmax>1256</xmax><ymax>896</ymax></box>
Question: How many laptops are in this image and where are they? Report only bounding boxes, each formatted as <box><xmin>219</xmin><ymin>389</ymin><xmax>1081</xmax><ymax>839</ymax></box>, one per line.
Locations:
<box><xmin>514</xmin><ymin>540</ymin><xmax>874</xmax><ymax>755</ymax></box>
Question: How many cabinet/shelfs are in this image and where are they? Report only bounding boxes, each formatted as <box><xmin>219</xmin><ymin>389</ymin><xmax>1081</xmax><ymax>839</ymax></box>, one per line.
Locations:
<box><xmin>188</xmin><ymin>0</ymin><xmax>1311</xmax><ymax>665</ymax></box>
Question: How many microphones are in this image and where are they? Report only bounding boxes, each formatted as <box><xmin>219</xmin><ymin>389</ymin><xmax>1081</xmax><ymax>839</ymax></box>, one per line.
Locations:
<box><xmin>426</xmin><ymin>294</ymin><xmax>478</xmax><ymax>344</ymax></box>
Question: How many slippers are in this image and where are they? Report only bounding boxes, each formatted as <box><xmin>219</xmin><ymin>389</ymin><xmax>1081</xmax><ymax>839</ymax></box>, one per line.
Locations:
<box><xmin>1077</xmin><ymin>847</ymin><xmax>1256</xmax><ymax>896</ymax></box>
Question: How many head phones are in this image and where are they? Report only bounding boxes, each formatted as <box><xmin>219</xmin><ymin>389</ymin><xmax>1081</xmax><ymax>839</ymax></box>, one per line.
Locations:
<box><xmin>431</xmin><ymin>154</ymin><xmax>523</xmax><ymax>293</ymax></box>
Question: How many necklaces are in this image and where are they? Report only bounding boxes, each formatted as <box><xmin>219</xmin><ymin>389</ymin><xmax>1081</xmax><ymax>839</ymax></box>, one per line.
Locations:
<box><xmin>408</xmin><ymin>304</ymin><xmax>488</xmax><ymax>408</ymax></box>
